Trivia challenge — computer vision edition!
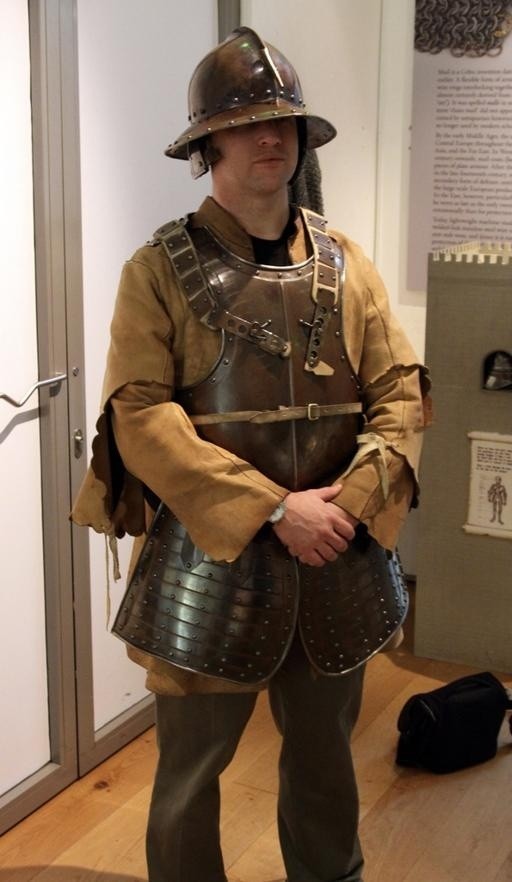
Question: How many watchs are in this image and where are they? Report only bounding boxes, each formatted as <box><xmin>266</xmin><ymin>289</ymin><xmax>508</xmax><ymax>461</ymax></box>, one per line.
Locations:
<box><xmin>268</xmin><ymin>501</ymin><xmax>285</xmax><ymax>523</ymax></box>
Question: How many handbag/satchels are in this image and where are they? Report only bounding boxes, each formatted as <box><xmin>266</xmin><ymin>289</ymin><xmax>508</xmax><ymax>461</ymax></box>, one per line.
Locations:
<box><xmin>388</xmin><ymin>668</ymin><xmax>512</xmax><ymax>774</ymax></box>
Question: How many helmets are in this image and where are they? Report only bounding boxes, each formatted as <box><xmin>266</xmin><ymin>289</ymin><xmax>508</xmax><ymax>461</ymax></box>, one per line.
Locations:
<box><xmin>159</xmin><ymin>22</ymin><xmax>335</xmax><ymax>161</ymax></box>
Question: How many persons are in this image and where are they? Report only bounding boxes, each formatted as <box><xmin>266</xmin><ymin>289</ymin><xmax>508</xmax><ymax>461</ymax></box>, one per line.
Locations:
<box><xmin>65</xmin><ymin>23</ymin><xmax>435</xmax><ymax>880</ymax></box>
<box><xmin>486</xmin><ymin>476</ymin><xmax>507</xmax><ymax>526</ymax></box>
